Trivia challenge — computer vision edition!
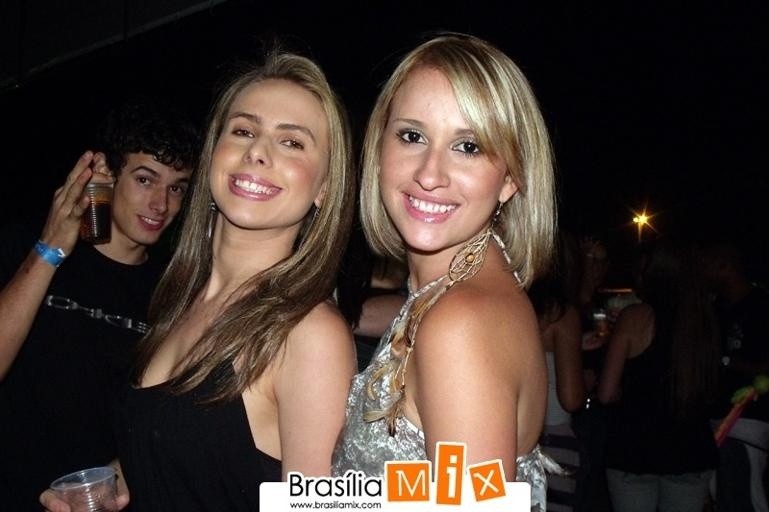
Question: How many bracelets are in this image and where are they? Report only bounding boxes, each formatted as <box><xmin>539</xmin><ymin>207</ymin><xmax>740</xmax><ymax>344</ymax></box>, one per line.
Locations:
<box><xmin>33</xmin><ymin>239</ymin><xmax>66</xmax><ymax>272</ymax></box>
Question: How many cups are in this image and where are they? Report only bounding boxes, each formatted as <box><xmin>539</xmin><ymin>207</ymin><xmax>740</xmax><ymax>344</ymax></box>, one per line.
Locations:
<box><xmin>48</xmin><ymin>465</ymin><xmax>121</xmax><ymax>512</ymax></box>
<box><xmin>592</xmin><ymin>310</ymin><xmax>609</xmax><ymax>338</ymax></box>
<box><xmin>77</xmin><ymin>178</ymin><xmax>116</xmax><ymax>244</ymax></box>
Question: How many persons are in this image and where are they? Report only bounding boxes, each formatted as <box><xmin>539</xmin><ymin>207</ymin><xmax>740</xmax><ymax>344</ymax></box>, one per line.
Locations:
<box><xmin>0</xmin><ymin>94</ymin><xmax>200</xmax><ymax>511</ymax></box>
<box><xmin>37</xmin><ymin>51</ymin><xmax>358</xmax><ymax>512</ymax></box>
<box><xmin>528</xmin><ymin>223</ymin><xmax>769</xmax><ymax>510</ymax></box>
<box><xmin>324</xmin><ymin>23</ymin><xmax>562</xmax><ymax>509</ymax></box>
